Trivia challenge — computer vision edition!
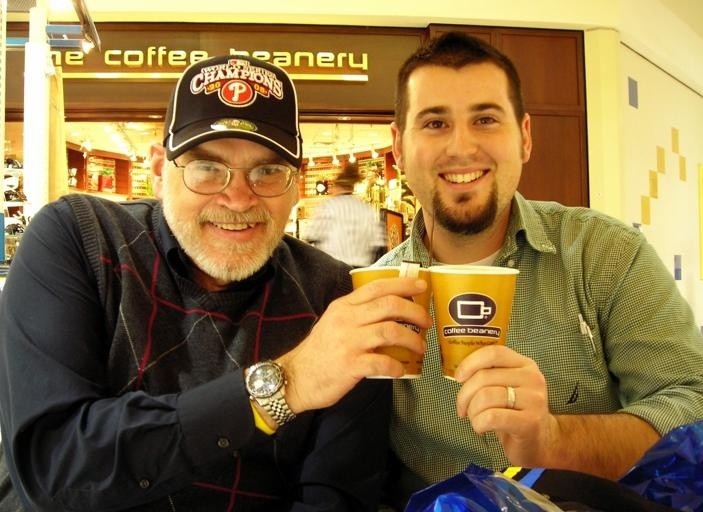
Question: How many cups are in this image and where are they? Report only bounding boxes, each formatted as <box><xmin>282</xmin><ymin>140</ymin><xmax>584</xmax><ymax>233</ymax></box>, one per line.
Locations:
<box><xmin>348</xmin><ymin>263</ymin><xmax>432</xmax><ymax>378</ymax></box>
<box><xmin>428</xmin><ymin>265</ymin><xmax>520</xmax><ymax>383</ymax></box>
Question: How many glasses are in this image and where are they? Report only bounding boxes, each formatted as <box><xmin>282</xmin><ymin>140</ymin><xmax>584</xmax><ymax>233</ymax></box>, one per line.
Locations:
<box><xmin>165</xmin><ymin>157</ymin><xmax>303</xmax><ymax>197</ymax></box>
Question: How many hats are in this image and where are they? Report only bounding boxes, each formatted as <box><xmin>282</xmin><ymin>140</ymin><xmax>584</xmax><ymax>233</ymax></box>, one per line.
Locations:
<box><xmin>162</xmin><ymin>54</ymin><xmax>305</xmax><ymax>170</ymax></box>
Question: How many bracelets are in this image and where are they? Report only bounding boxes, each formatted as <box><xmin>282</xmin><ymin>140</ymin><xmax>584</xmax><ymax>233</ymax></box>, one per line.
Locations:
<box><xmin>245</xmin><ymin>398</ymin><xmax>280</xmax><ymax>440</ymax></box>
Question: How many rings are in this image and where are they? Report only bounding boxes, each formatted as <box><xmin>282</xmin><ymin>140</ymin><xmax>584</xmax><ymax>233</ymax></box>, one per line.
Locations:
<box><xmin>506</xmin><ymin>385</ymin><xmax>516</xmax><ymax>410</ymax></box>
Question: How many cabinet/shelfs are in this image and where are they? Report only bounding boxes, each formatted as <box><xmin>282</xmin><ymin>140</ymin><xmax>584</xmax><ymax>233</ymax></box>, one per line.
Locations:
<box><xmin>289</xmin><ymin>144</ymin><xmax>401</xmax><ymax>253</ymax></box>
<box><xmin>65</xmin><ymin>142</ymin><xmax>153</xmax><ymax>203</ymax></box>
<box><xmin>2</xmin><ymin>149</ymin><xmax>25</xmax><ymax>264</ymax></box>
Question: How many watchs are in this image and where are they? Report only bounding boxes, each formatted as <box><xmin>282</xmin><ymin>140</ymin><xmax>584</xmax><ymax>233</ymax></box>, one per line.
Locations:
<box><xmin>244</xmin><ymin>356</ymin><xmax>298</xmax><ymax>427</ymax></box>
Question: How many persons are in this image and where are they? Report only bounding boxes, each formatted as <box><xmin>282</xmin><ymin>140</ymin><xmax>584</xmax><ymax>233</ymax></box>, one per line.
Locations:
<box><xmin>353</xmin><ymin>29</ymin><xmax>703</xmax><ymax>511</ymax></box>
<box><xmin>1</xmin><ymin>55</ymin><xmax>434</xmax><ymax>511</ymax></box>
<box><xmin>299</xmin><ymin>170</ymin><xmax>388</xmax><ymax>273</ymax></box>
